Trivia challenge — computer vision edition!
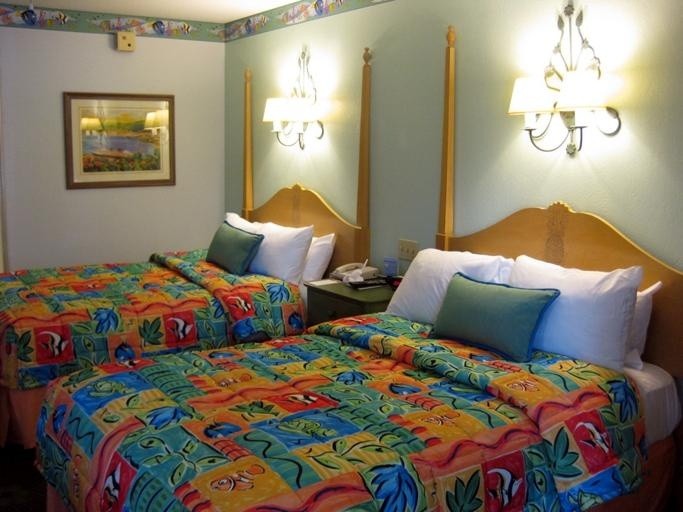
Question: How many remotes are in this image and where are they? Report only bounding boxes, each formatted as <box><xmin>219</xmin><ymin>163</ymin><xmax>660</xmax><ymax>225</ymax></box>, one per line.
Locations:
<box><xmin>349</xmin><ymin>279</ymin><xmax>386</xmax><ymax>291</ymax></box>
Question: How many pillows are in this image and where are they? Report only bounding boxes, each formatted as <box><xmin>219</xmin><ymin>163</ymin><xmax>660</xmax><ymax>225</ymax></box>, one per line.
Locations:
<box><xmin>206</xmin><ymin>210</ymin><xmax>336</xmax><ymax>309</ymax></box>
<box><xmin>385</xmin><ymin>248</ymin><xmax>661</xmax><ymax>376</ymax></box>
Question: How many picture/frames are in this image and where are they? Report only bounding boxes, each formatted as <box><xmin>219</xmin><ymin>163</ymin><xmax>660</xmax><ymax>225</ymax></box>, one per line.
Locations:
<box><xmin>64</xmin><ymin>91</ymin><xmax>176</xmax><ymax>188</ymax></box>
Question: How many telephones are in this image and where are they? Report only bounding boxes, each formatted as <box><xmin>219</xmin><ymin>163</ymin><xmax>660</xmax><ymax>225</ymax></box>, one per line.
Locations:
<box><xmin>332</xmin><ymin>262</ymin><xmax>379</xmax><ymax>281</ymax></box>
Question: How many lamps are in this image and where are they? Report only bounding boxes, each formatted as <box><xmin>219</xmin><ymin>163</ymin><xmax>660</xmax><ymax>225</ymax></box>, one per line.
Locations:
<box><xmin>511</xmin><ymin>9</ymin><xmax>621</xmax><ymax>160</ymax></box>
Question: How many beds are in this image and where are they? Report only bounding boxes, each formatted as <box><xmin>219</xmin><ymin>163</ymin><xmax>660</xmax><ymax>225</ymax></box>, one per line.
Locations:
<box><xmin>0</xmin><ymin>183</ymin><xmax>364</xmax><ymax>449</ymax></box>
<box><xmin>41</xmin><ymin>200</ymin><xmax>682</xmax><ymax>510</ymax></box>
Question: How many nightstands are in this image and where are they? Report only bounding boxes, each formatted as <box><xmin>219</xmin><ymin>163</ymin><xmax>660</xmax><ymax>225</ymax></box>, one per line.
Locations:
<box><xmin>304</xmin><ymin>279</ymin><xmax>395</xmax><ymax>329</ymax></box>
